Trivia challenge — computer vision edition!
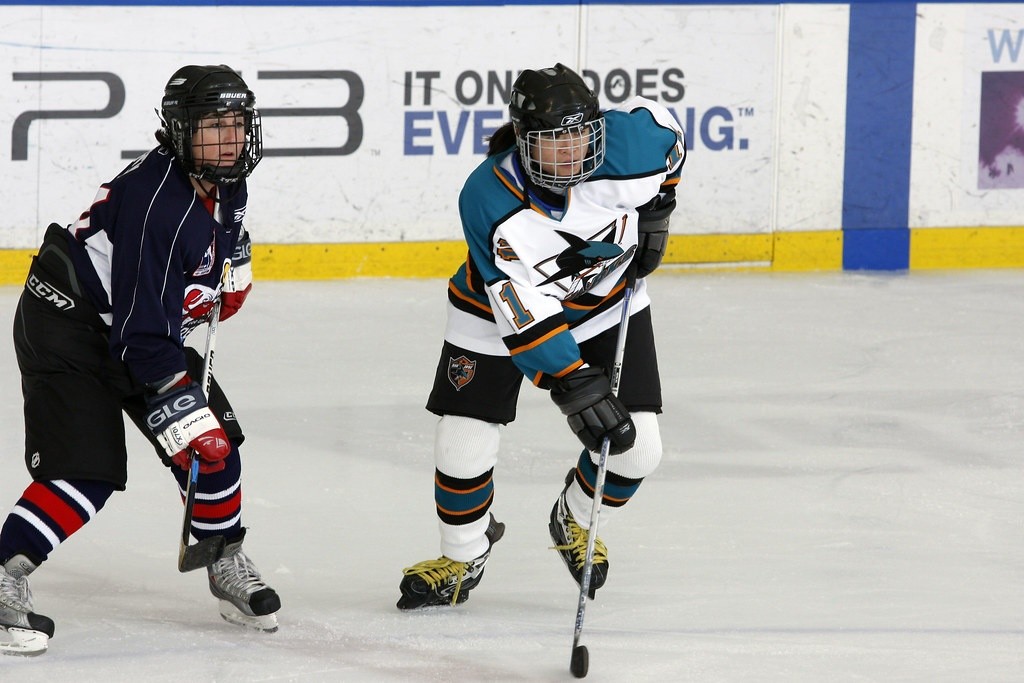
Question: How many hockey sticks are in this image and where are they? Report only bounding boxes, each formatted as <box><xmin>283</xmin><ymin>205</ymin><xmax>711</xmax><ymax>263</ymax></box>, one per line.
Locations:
<box><xmin>173</xmin><ymin>293</ymin><xmax>228</xmax><ymax>574</ymax></box>
<box><xmin>569</xmin><ymin>262</ymin><xmax>643</xmax><ymax>680</ymax></box>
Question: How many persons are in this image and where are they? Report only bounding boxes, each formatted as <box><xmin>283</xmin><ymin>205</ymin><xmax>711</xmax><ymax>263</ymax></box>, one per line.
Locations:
<box><xmin>394</xmin><ymin>62</ymin><xmax>686</xmax><ymax>612</ymax></box>
<box><xmin>0</xmin><ymin>62</ymin><xmax>282</xmax><ymax>657</ymax></box>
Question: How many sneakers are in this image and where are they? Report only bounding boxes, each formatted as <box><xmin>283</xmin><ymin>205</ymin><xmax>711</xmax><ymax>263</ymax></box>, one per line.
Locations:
<box><xmin>0</xmin><ymin>550</ymin><xmax>55</xmax><ymax>657</ymax></box>
<box><xmin>199</xmin><ymin>526</ymin><xmax>281</xmax><ymax>634</ymax></box>
<box><xmin>397</xmin><ymin>512</ymin><xmax>505</xmax><ymax>611</ymax></box>
<box><xmin>548</xmin><ymin>468</ymin><xmax>609</xmax><ymax>600</ymax></box>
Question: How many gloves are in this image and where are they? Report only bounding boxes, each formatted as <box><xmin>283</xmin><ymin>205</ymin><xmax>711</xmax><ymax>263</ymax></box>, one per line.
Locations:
<box><xmin>184</xmin><ymin>231</ymin><xmax>254</xmax><ymax>322</ymax></box>
<box><xmin>142</xmin><ymin>372</ymin><xmax>230</xmax><ymax>474</ymax></box>
<box><xmin>633</xmin><ymin>188</ymin><xmax>676</xmax><ymax>279</ymax></box>
<box><xmin>550</xmin><ymin>367</ymin><xmax>636</xmax><ymax>457</ymax></box>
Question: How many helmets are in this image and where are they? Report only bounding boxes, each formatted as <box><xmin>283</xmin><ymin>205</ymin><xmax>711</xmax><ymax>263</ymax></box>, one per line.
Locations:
<box><xmin>509</xmin><ymin>62</ymin><xmax>606</xmax><ymax>192</ymax></box>
<box><xmin>160</xmin><ymin>64</ymin><xmax>264</xmax><ymax>188</ymax></box>
